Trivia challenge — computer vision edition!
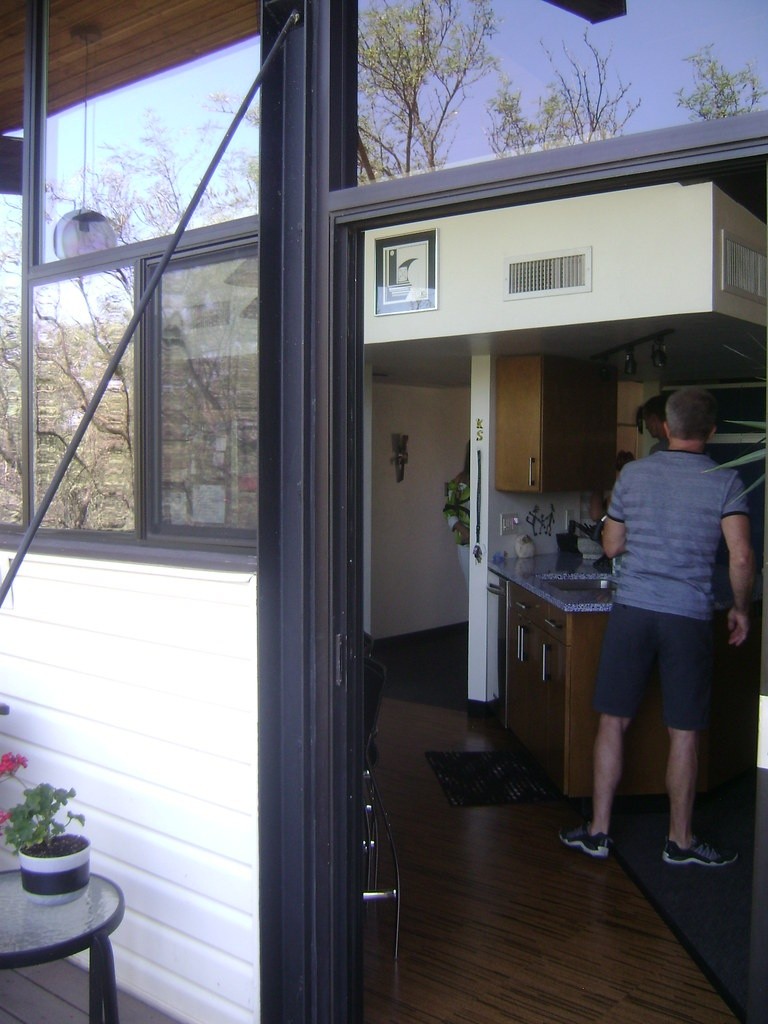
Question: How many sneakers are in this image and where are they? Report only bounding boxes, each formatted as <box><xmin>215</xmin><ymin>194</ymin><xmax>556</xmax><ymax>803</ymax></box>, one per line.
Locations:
<box><xmin>660</xmin><ymin>832</ymin><xmax>739</xmax><ymax>867</ymax></box>
<box><xmin>558</xmin><ymin>820</ymin><xmax>614</xmax><ymax>859</ymax></box>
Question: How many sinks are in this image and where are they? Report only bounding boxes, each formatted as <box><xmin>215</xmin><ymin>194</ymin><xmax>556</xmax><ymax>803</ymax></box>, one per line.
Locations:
<box><xmin>541</xmin><ymin>576</ymin><xmax>626</xmax><ymax>595</ymax></box>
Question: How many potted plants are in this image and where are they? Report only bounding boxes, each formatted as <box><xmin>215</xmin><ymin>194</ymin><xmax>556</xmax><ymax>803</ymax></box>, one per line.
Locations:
<box><xmin>26</xmin><ymin>829</ymin><xmax>91</xmax><ymax>906</ymax></box>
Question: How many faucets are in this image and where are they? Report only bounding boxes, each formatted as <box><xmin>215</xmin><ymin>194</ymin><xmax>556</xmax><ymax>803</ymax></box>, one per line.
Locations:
<box><xmin>588</xmin><ymin>514</ymin><xmax>610</xmax><ymax>541</ymax></box>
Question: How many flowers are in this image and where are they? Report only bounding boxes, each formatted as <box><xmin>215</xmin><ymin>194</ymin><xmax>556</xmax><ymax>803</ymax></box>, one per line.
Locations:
<box><xmin>0</xmin><ymin>751</ymin><xmax>87</xmax><ymax>850</ymax></box>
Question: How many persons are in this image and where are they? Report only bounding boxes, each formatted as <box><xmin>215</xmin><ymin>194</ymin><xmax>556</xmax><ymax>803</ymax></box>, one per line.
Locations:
<box><xmin>590</xmin><ymin>450</ymin><xmax>636</xmax><ymax>520</ymax></box>
<box><xmin>446</xmin><ymin>470</ymin><xmax>470</xmax><ymax>577</ymax></box>
<box><xmin>642</xmin><ymin>394</ymin><xmax>669</xmax><ymax>456</ymax></box>
<box><xmin>557</xmin><ymin>386</ymin><xmax>754</xmax><ymax>864</ymax></box>
